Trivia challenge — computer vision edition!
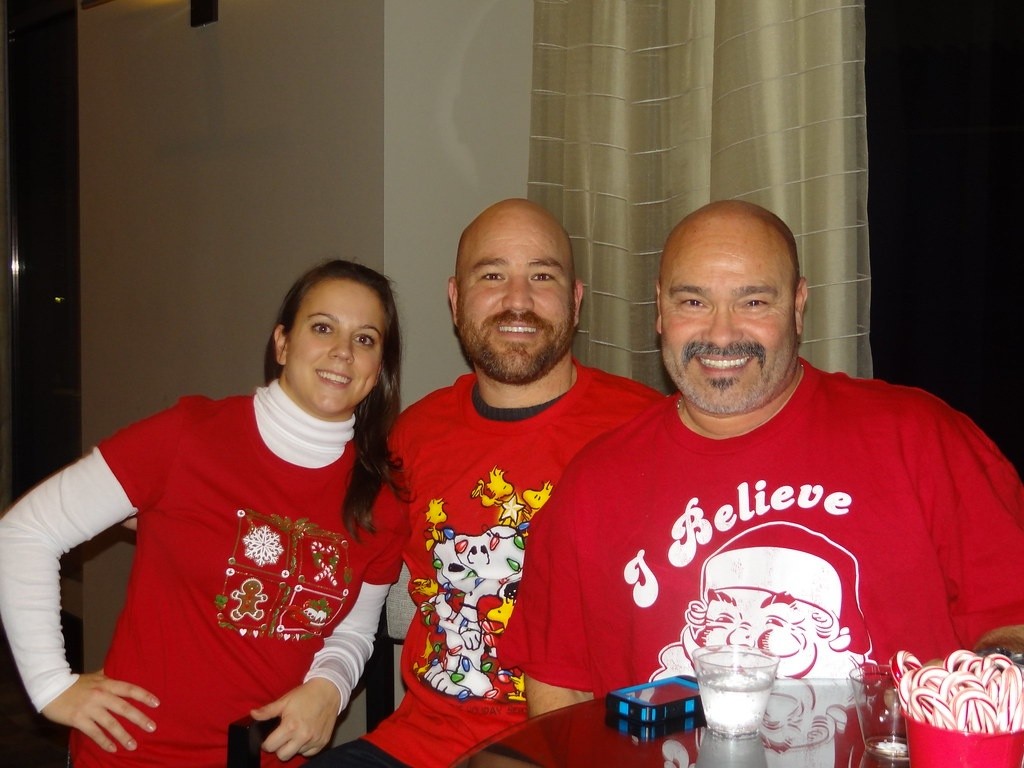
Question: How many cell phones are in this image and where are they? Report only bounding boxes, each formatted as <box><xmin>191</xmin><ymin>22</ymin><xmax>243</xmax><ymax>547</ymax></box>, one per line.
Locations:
<box><xmin>605</xmin><ymin>714</ymin><xmax>706</xmax><ymax>740</ymax></box>
<box><xmin>605</xmin><ymin>675</ymin><xmax>703</xmax><ymax>721</ymax></box>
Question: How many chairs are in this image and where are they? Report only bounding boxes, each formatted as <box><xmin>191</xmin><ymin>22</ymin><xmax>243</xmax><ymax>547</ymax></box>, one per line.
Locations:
<box><xmin>227</xmin><ymin>562</ymin><xmax>419</xmax><ymax>768</ymax></box>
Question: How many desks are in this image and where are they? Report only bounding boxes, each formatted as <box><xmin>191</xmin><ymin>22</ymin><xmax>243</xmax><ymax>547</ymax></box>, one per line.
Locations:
<box><xmin>438</xmin><ymin>668</ymin><xmax>908</xmax><ymax>768</ymax></box>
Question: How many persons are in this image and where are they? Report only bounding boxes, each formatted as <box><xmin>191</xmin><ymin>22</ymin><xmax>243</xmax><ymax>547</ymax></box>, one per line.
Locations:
<box><xmin>1</xmin><ymin>257</ymin><xmax>409</xmax><ymax>768</ymax></box>
<box><xmin>121</xmin><ymin>200</ymin><xmax>671</xmax><ymax>768</ymax></box>
<box><xmin>524</xmin><ymin>204</ymin><xmax>1023</xmax><ymax>768</ymax></box>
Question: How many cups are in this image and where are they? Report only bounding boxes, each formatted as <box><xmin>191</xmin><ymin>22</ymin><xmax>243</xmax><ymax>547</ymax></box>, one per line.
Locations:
<box><xmin>695</xmin><ymin>731</ymin><xmax>767</xmax><ymax>768</ymax></box>
<box><xmin>902</xmin><ymin>710</ymin><xmax>1024</xmax><ymax>768</ymax></box>
<box><xmin>691</xmin><ymin>644</ymin><xmax>780</xmax><ymax>739</ymax></box>
<box><xmin>848</xmin><ymin>665</ymin><xmax>908</xmax><ymax>759</ymax></box>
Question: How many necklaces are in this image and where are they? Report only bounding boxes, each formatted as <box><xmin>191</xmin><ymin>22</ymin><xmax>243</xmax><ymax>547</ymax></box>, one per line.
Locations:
<box><xmin>677</xmin><ymin>364</ymin><xmax>804</xmax><ymax>408</ymax></box>
